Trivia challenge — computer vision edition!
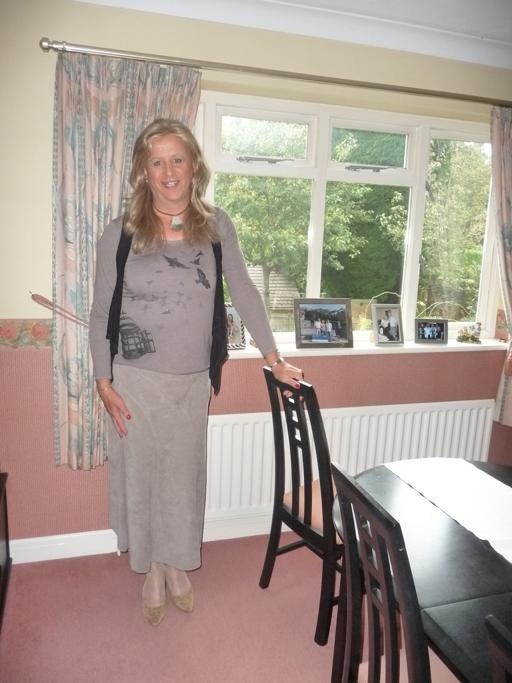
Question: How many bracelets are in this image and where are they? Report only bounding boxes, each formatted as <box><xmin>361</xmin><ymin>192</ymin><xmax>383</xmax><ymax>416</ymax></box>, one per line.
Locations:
<box><xmin>99</xmin><ymin>384</ymin><xmax>110</xmax><ymax>396</ymax></box>
<box><xmin>271</xmin><ymin>358</ymin><xmax>284</xmax><ymax>366</ymax></box>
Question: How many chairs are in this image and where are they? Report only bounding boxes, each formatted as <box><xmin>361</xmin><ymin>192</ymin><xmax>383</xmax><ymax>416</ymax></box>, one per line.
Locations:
<box><xmin>259</xmin><ymin>366</ymin><xmax>346</xmax><ymax>647</ymax></box>
<box><xmin>329</xmin><ymin>462</ymin><xmax>432</xmax><ymax>683</ymax></box>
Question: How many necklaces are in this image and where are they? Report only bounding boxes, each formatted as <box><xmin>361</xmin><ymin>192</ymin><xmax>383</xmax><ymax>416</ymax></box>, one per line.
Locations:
<box><xmin>152</xmin><ymin>202</ymin><xmax>192</xmax><ymax>232</ymax></box>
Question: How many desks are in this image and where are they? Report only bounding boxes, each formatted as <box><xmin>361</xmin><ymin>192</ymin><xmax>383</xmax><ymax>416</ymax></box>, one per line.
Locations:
<box><xmin>329</xmin><ymin>450</ymin><xmax>512</xmax><ymax>681</ymax></box>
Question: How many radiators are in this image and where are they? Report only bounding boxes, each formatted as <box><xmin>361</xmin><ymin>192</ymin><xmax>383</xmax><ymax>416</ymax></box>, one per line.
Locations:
<box><xmin>191</xmin><ymin>398</ymin><xmax>495</xmax><ymax>528</ymax></box>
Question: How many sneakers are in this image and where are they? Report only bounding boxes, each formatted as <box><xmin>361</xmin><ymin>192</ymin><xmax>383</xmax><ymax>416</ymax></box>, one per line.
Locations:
<box><xmin>142</xmin><ymin>586</ymin><xmax>196</xmax><ymax>629</ymax></box>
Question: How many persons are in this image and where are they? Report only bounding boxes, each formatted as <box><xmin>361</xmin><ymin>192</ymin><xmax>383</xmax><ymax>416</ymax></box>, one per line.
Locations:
<box><xmin>225</xmin><ymin>308</ymin><xmax>241</xmax><ymax>343</ymax></box>
<box><xmin>89</xmin><ymin>120</ymin><xmax>302</xmax><ymax>626</ymax></box>
<box><xmin>379</xmin><ymin>310</ymin><xmax>398</xmax><ymax>341</ymax></box>
<box><xmin>315</xmin><ymin>318</ymin><xmax>332</xmax><ymax>342</ymax></box>
<box><xmin>419</xmin><ymin>323</ymin><xmax>442</xmax><ymax>339</ymax></box>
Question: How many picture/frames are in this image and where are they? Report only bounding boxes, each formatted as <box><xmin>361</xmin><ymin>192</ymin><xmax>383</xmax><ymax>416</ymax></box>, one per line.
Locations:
<box><xmin>293</xmin><ymin>298</ymin><xmax>450</xmax><ymax>348</ymax></box>
<box><xmin>222</xmin><ymin>302</ymin><xmax>246</xmax><ymax>349</ymax></box>
